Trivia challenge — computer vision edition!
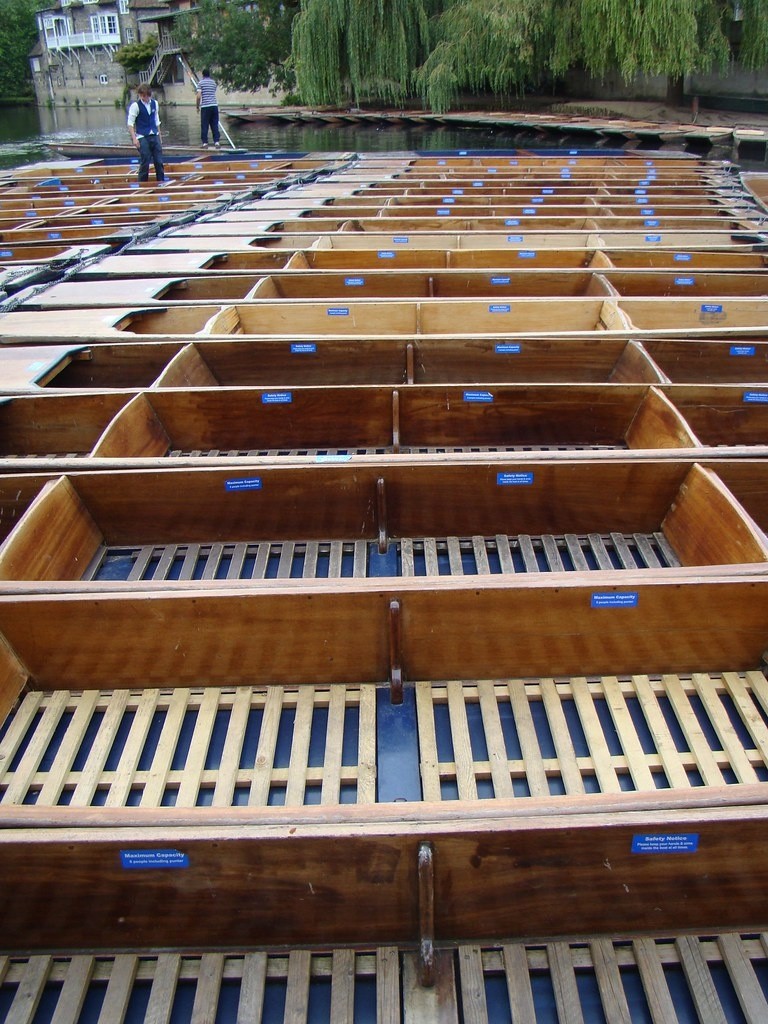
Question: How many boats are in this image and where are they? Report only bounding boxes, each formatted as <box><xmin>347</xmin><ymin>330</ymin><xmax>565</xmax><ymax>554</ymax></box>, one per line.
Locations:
<box><xmin>43</xmin><ymin>141</ymin><xmax>246</xmax><ymax>158</ymax></box>
<box><xmin>0</xmin><ymin>150</ymin><xmax>768</xmax><ymax>1024</ymax></box>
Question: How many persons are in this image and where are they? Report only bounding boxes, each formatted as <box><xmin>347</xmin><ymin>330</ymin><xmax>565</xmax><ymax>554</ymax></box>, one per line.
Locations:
<box><xmin>127</xmin><ymin>84</ymin><xmax>165</xmax><ymax>182</ymax></box>
<box><xmin>195</xmin><ymin>68</ymin><xmax>221</xmax><ymax>149</ymax></box>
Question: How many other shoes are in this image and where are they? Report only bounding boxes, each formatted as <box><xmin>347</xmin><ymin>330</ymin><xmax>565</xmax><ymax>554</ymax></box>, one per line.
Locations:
<box><xmin>216</xmin><ymin>144</ymin><xmax>221</xmax><ymax>149</ymax></box>
<box><xmin>199</xmin><ymin>145</ymin><xmax>208</xmax><ymax>149</ymax></box>
